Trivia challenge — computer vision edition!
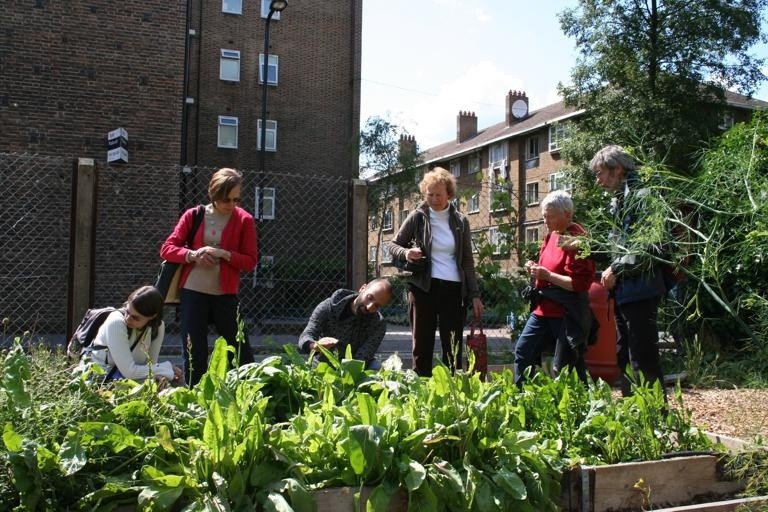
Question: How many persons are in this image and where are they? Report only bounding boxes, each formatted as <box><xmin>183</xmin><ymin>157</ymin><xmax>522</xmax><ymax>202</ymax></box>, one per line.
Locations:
<box><xmin>389</xmin><ymin>167</ymin><xmax>485</xmax><ymax>378</ymax></box>
<box><xmin>513</xmin><ymin>190</ymin><xmax>596</xmax><ymax>390</ymax></box>
<box><xmin>296</xmin><ymin>279</ymin><xmax>391</xmax><ymax>371</ymax></box>
<box><xmin>590</xmin><ymin>146</ymin><xmax>678</xmax><ymax>420</ymax></box>
<box><xmin>159</xmin><ymin>168</ymin><xmax>257</xmax><ymax>389</ymax></box>
<box><xmin>66</xmin><ymin>287</ymin><xmax>183</xmax><ymax>386</ymax></box>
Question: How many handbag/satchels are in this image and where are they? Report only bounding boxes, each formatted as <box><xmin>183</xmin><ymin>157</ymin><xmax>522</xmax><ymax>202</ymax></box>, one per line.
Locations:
<box><xmin>154</xmin><ymin>260</ymin><xmax>184</xmax><ymax>306</ymax></box>
<box><xmin>466</xmin><ymin>322</ymin><xmax>487</xmax><ymax>382</ymax></box>
<box><xmin>391</xmin><ymin>243</ymin><xmax>427</xmax><ymax>273</ymax></box>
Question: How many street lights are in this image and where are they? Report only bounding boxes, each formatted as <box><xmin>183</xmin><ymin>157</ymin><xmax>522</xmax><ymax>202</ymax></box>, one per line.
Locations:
<box><xmin>256</xmin><ymin>0</ymin><xmax>287</xmax><ymax>334</ymax></box>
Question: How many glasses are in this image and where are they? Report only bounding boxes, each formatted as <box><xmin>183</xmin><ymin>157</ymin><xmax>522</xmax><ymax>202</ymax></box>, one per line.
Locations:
<box><xmin>122</xmin><ymin>301</ymin><xmax>140</xmax><ymax>322</ymax></box>
<box><xmin>221</xmin><ymin>198</ymin><xmax>242</xmax><ymax>203</ymax></box>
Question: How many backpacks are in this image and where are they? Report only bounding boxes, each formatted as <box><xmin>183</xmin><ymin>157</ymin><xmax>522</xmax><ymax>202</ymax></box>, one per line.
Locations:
<box><xmin>67</xmin><ymin>307</ymin><xmax>158</xmax><ymax>360</ymax></box>
